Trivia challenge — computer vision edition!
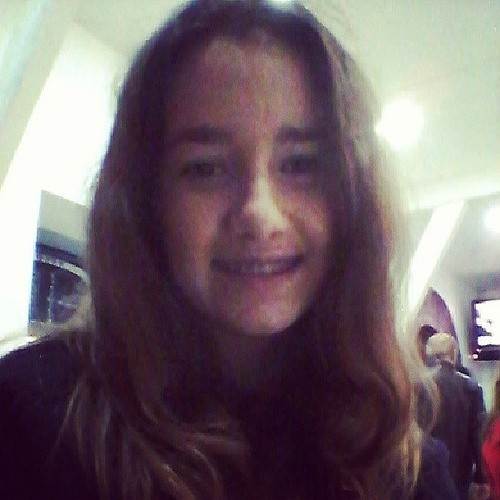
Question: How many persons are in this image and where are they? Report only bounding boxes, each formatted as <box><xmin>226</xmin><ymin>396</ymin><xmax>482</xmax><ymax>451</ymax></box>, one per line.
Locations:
<box><xmin>407</xmin><ymin>325</ymin><xmax>500</xmax><ymax>500</ymax></box>
<box><xmin>0</xmin><ymin>0</ymin><xmax>441</xmax><ymax>500</ymax></box>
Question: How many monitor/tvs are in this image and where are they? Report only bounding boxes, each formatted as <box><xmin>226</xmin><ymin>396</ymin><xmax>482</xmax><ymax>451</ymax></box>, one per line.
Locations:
<box><xmin>471</xmin><ymin>298</ymin><xmax>500</xmax><ymax>361</ymax></box>
